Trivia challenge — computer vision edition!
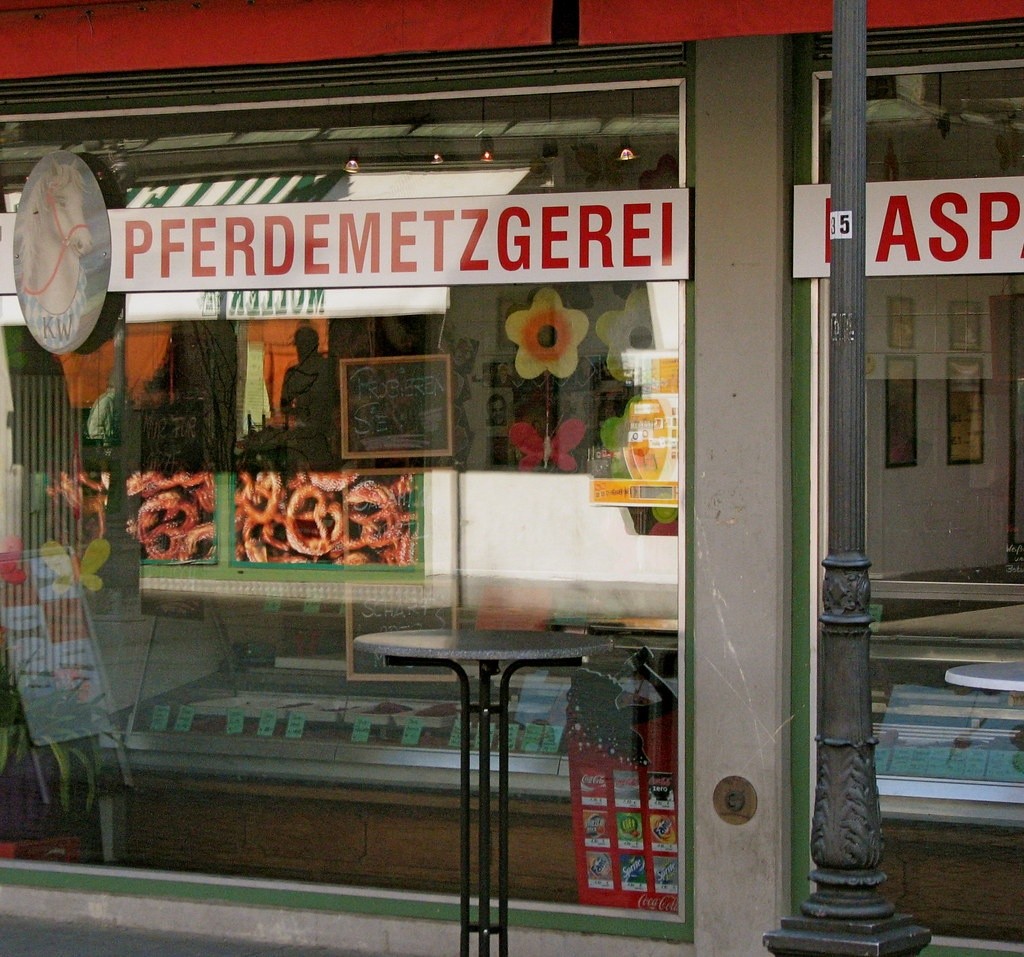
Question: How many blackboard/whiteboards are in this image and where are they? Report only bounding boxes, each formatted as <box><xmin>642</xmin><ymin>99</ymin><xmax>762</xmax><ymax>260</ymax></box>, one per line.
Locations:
<box><xmin>339</xmin><ymin>352</ymin><xmax>454</xmax><ymax>459</ymax></box>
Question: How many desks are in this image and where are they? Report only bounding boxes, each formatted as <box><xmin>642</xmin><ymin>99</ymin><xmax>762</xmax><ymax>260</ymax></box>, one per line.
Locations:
<box><xmin>354</xmin><ymin>627</ymin><xmax>616</xmax><ymax>957</ymax></box>
<box><xmin>944</xmin><ymin>661</ymin><xmax>1024</xmax><ymax>692</ymax></box>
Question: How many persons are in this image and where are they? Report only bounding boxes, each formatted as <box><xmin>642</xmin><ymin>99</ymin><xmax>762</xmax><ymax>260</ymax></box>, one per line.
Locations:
<box><xmin>483</xmin><ymin>358</ymin><xmax>520</xmax><ymax>428</ymax></box>
<box><xmin>280</xmin><ymin>325</ymin><xmax>336</xmax><ymax>436</ymax></box>
<box><xmin>86</xmin><ymin>373</ymin><xmax>119</xmax><ymax>490</ymax></box>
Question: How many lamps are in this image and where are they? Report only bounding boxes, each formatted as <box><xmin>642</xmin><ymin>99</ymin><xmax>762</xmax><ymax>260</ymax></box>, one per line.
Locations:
<box><xmin>479</xmin><ymin>137</ymin><xmax>495</xmax><ymax>162</ymax></box>
<box><xmin>612</xmin><ymin>137</ymin><xmax>641</xmax><ymax>162</ymax></box>
<box><xmin>344</xmin><ymin>146</ymin><xmax>361</xmax><ymax>174</ymax></box>
<box><xmin>428</xmin><ymin>140</ymin><xmax>444</xmax><ymax>166</ymax></box>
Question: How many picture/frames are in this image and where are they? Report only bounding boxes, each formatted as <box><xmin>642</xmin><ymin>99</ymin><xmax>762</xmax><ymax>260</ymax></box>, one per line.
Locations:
<box><xmin>946</xmin><ymin>357</ymin><xmax>986</xmax><ymax>465</ymax></box>
<box><xmin>338</xmin><ymin>353</ymin><xmax>455</xmax><ymax>459</ymax></box>
<box><xmin>947</xmin><ymin>298</ymin><xmax>983</xmax><ymax>352</ymax></box>
<box><xmin>885</xmin><ymin>295</ymin><xmax>915</xmax><ymax>350</ymax></box>
<box><xmin>884</xmin><ymin>352</ymin><xmax>919</xmax><ymax>470</ymax></box>
